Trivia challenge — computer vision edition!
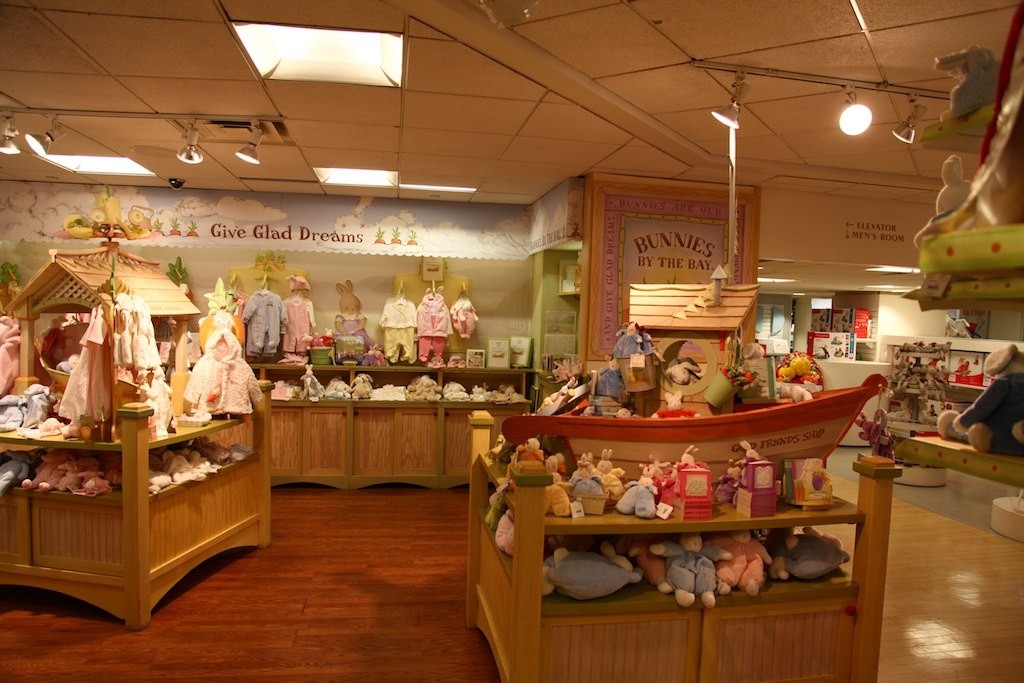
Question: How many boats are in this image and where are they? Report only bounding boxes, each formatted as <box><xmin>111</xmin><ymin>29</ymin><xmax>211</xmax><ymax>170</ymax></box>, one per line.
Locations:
<box><xmin>501</xmin><ymin>373</ymin><xmax>889</xmax><ymax>503</ymax></box>
<box><xmin>38</xmin><ymin>321</ymin><xmax>144</xmax><ymax>406</ymax></box>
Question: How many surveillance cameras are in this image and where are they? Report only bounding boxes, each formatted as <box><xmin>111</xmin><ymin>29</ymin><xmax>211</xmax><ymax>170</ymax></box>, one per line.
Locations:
<box><xmin>167</xmin><ymin>177</ymin><xmax>184</xmax><ymax>190</ymax></box>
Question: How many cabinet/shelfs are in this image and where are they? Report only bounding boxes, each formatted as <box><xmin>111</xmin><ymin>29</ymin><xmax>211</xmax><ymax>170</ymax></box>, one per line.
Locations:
<box><xmin>0</xmin><ymin>378</ymin><xmax>276</xmax><ymax>631</ymax></box>
<box><xmin>879</xmin><ymin>337</ymin><xmax>1024</xmax><ymax>432</ymax></box>
<box><xmin>248</xmin><ymin>362</ymin><xmax>535</xmax><ymax>490</ymax></box>
<box><xmin>466</xmin><ymin>412</ymin><xmax>903</xmax><ymax>683</ymax></box>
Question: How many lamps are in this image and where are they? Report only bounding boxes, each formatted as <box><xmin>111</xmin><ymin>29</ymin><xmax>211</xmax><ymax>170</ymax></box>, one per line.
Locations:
<box><xmin>892</xmin><ymin>93</ymin><xmax>927</xmax><ymax>144</ymax></box>
<box><xmin>712</xmin><ymin>71</ymin><xmax>749</xmax><ymax>131</ymax></box>
<box><xmin>0</xmin><ymin>110</ymin><xmax>21</xmax><ymax>155</ymax></box>
<box><xmin>235</xmin><ymin>120</ymin><xmax>265</xmax><ymax>165</ymax></box>
<box><xmin>175</xmin><ymin>118</ymin><xmax>204</xmax><ymax>165</ymax></box>
<box><xmin>838</xmin><ymin>86</ymin><xmax>873</xmax><ymax>135</ymax></box>
<box><xmin>25</xmin><ymin>113</ymin><xmax>67</xmax><ymax>158</ymax></box>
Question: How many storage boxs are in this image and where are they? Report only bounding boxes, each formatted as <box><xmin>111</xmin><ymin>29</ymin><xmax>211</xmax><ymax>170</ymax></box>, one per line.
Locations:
<box><xmin>810</xmin><ymin>308</ymin><xmax>869</xmax><ymax>339</ymax></box>
<box><xmin>927</xmin><ymin>398</ymin><xmax>975</xmax><ymax>418</ymax></box>
<box><xmin>807</xmin><ymin>331</ymin><xmax>857</xmax><ymax>364</ymax></box>
<box><xmin>947</xmin><ymin>352</ymin><xmax>983</xmax><ymax>386</ymax></box>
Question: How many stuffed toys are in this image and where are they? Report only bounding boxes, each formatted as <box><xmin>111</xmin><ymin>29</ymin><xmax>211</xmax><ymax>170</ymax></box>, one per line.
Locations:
<box><xmin>934</xmin><ymin>44</ymin><xmax>1000</xmax><ymax>214</ymax></box>
<box><xmin>889</xmin><ymin>341</ymin><xmax>1024</xmax><ymax>459</ymax></box>
<box><xmin>300</xmin><ymin>364</ymin><xmax>531</xmax><ymax>404</ymax></box>
<box><xmin>1</xmin><ymin>356</ymin><xmax>234</xmax><ymax>495</ymax></box>
<box><xmin>484</xmin><ymin>321</ymin><xmax>851</xmax><ymax>607</ymax></box>
